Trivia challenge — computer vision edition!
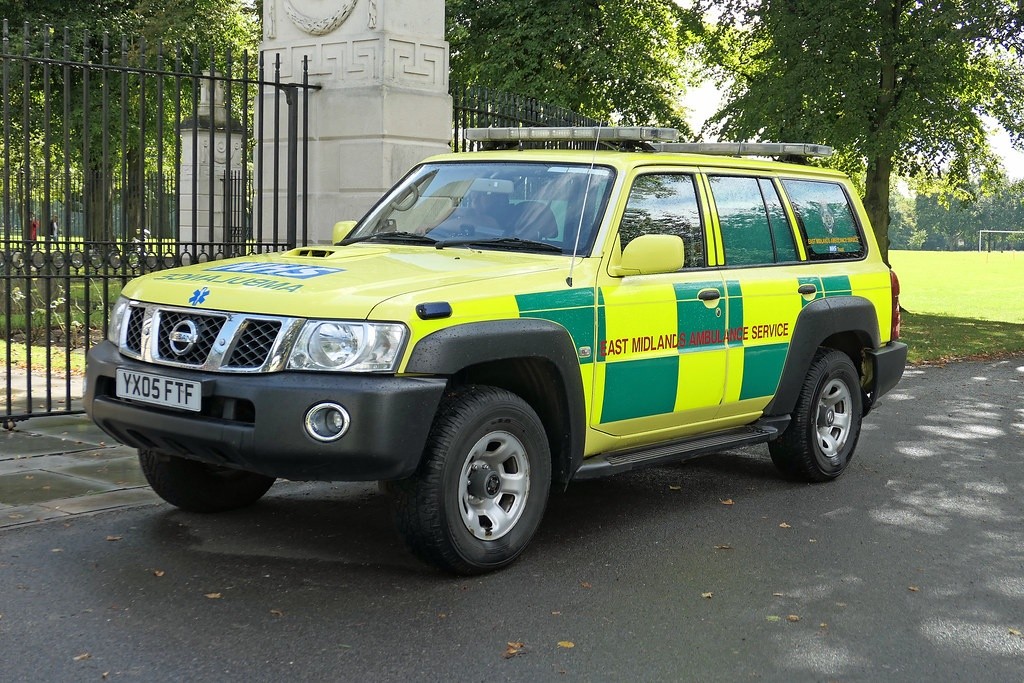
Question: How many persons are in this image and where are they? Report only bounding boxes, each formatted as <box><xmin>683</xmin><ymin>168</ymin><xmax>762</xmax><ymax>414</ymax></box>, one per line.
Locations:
<box><xmin>470</xmin><ymin>178</ymin><xmax>510</xmax><ymax>232</ymax></box>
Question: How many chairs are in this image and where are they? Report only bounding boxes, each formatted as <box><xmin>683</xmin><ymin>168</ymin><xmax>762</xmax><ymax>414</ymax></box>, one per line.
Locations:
<box><xmin>512</xmin><ymin>201</ymin><xmax>561</xmax><ymax>246</ymax></box>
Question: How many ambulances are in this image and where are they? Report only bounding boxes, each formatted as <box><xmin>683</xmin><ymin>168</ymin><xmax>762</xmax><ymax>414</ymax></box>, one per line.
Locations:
<box><xmin>80</xmin><ymin>124</ymin><xmax>911</xmax><ymax>578</ymax></box>
